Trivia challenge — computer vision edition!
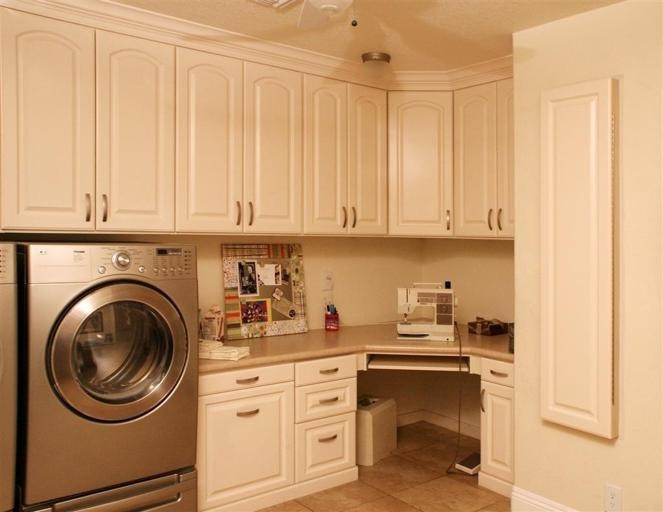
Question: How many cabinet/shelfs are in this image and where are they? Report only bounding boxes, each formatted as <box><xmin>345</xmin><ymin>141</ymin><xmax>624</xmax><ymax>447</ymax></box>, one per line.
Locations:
<box><xmin>175</xmin><ymin>18</ymin><xmax>302</xmax><ymax>236</ymax></box>
<box><xmin>1</xmin><ymin>0</ymin><xmax>176</xmax><ymax>232</ymax></box>
<box><xmin>294</xmin><ymin>353</ymin><xmax>359</xmax><ymax>500</ymax></box>
<box><xmin>302</xmin><ymin>49</ymin><xmax>388</xmax><ymax>239</ymax></box>
<box><xmin>386</xmin><ymin>70</ymin><xmax>455</xmax><ymax>239</ymax></box>
<box><xmin>452</xmin><ymin>54</ymin><xmax>514</xmax><ymax>242</ymax></box>
<box><xmin>197</xmin><ymin>364</ymin><xmax>294</xmax><ymax>512</ymax></box>
<box><xmin>478</xmin><ymin>358</ymin><xmax>515</xmax><ymax>500</ymax></box>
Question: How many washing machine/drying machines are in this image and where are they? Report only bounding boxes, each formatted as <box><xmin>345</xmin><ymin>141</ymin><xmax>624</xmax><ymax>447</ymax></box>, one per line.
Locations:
<box><xmin>17</xmin><ymin>242</ymin><xmax>198</xmax><ymax>512</ymax></box>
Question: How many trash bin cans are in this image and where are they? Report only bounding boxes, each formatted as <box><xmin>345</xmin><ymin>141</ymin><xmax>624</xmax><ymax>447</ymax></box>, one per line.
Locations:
<box><xmin>357</xmin><ymin>397</ymin><xmax>398</xmax><ymax>467</ymax></box>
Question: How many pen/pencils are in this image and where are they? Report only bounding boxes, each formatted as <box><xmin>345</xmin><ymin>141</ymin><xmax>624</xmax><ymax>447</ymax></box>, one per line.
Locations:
<box><xmin>326</xmin><ymin>303</ymin><xmax>336</xmax><ymax>314</ymax></box>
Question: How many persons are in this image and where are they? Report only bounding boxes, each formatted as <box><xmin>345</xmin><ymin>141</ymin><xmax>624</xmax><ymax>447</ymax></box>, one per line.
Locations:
<box><xmin>247</xmin><ymin>264</ymin><xmax>255</xmax><ymax>282</ymax></box>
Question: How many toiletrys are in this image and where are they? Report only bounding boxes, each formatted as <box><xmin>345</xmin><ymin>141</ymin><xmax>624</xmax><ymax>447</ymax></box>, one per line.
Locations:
<box><xmin>325</xmin><ymin>304</ymin><xmax>340</xmax><ymax>330</ymax></box>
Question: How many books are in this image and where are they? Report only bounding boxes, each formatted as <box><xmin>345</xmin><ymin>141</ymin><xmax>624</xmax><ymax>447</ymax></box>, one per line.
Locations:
<box><xmin>454</xmin><ymin>452</ymin><xmax>481</xmax><ymax>476</ymax></box>
<box><xmin>225</xmin><ymin>328</ymin><xmax>241</xmax><ymax>340</ymax></box>
<box><xmin>198</xmin><ymin>338</ymin><xmax>223</xmax><ymax>351</ymax></box>
<box><xmin>199</xmin><ymin>346</ymin><xmax>251</xmax><ymax>362</ymax></box>
